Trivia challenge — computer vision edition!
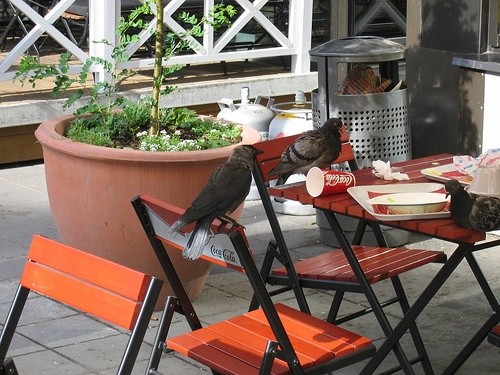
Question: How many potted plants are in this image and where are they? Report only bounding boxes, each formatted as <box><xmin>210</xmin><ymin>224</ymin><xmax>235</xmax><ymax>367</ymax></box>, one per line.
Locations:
<box><xmin>12</xmin><ymin>0</ymin><xmax>264</xmax><ymax>314</ymax></box>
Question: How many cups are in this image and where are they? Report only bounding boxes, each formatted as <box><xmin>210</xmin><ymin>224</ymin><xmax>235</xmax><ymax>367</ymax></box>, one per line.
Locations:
<box><xmin>305</xmin><ymin>166</ymin><xmax>357</xmax><ymax>199</ymax></box>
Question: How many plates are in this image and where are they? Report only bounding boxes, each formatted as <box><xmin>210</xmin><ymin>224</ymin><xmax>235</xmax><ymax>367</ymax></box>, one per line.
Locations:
<box><xmin>365</xmin><ymin>191</ymin><xmax>450</xmax><ymax>215</ymax></box>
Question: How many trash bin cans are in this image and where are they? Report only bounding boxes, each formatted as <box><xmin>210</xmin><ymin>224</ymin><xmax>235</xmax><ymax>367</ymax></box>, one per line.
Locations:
<box><xmin>308</xmin><ymin>35</ymin><xmax>412</xmax><ymax>248</ymax></box>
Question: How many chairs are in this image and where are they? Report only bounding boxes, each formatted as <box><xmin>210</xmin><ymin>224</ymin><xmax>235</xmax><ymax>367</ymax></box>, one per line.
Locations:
<box><xmin>0</xmin><ymin>231</ymin><xmax>164</xmax><ymax>375</ymax></box>
<box><xmin>244</xmin><ymin>122</ymin><xmax>449</xmax><ymax>374</ymax></box>
<box><xmin>129</xmin><ymin>191</ymin><xmax>376</xmax><ymax>374</ymax></box>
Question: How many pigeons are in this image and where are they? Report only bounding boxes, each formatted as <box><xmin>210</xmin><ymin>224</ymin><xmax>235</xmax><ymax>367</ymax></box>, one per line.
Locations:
<box><xmin>445</xmin><ymin>179</ymin><xmax>500</xmax><ymax>232</ymax></box>
<box><xmin>267</xmin><ymin>118</ymin><xmax>343</xmax><ymax>186</ymax></box>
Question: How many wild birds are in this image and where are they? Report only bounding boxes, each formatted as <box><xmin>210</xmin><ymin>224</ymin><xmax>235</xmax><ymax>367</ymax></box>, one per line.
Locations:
<box><xmin>168</xmin><ymin>144</ymin><xmax>264</xmax><ymax>261</ymax></box>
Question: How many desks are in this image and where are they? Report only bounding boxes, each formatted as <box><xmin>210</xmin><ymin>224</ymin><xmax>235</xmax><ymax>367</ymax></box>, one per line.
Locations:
<box><xmin>264</xmin><ymin>151</ymin><xmax>499</xmax><ymax>374</ymax></box>
